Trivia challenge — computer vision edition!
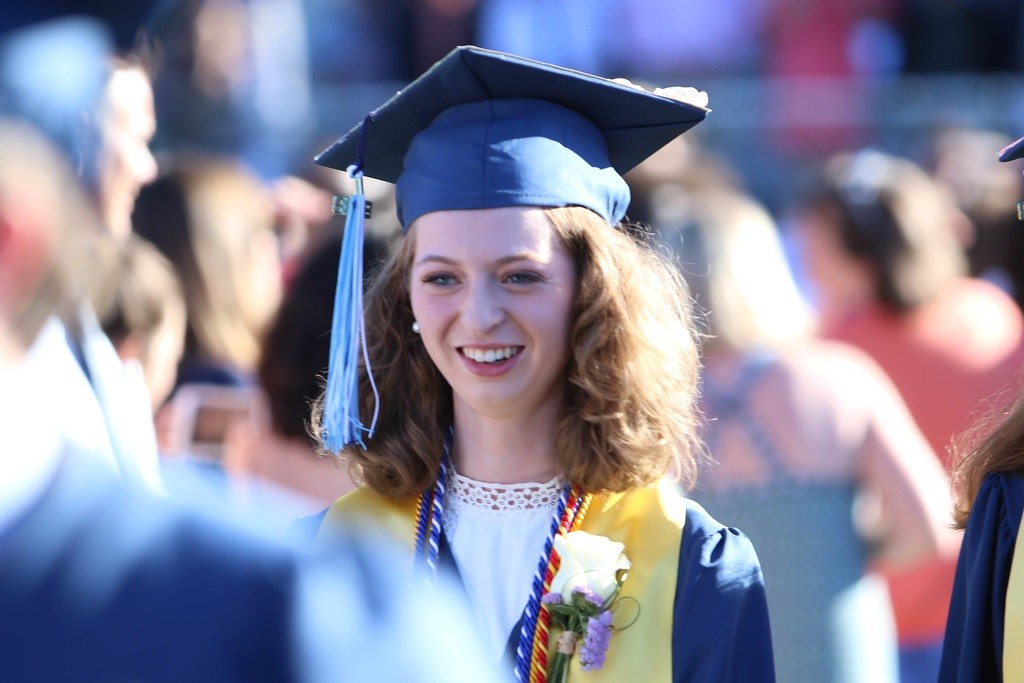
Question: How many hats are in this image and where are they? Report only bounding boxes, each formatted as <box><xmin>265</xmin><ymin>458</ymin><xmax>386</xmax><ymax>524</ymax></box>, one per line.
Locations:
<box><xmin>999</xmin><ymin>135</ymin><xmax>1024</xmax><ymax>163</ymax></box>
<box><xmin>313</xmin><ymin>45</ymin><xmax>712</xmax><ymax>454</ymax></box>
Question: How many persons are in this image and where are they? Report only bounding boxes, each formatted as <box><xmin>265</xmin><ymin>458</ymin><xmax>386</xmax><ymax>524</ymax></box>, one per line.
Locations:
<box><xmin>0</xmin><ymin>0</ymin><xmax>1024</xmax><ymax>683</ymax></box>
<box><xmin>293</xmin><ymin>45</ymin><xmax>777</xmax><ymax>683</ymax></box>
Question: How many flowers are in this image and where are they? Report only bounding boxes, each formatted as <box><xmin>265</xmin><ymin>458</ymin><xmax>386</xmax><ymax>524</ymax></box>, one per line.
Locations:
<box><xmin>541</xmin><ymin>528</ymin><xmax>641</xmax><ymax>683</ymax></box>
